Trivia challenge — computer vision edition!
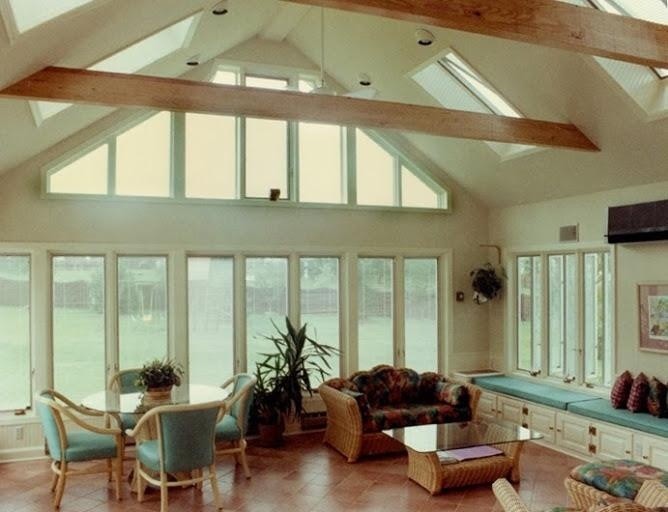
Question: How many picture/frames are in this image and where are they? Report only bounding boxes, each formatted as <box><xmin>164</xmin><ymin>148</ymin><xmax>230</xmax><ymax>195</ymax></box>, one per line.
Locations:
<box><xmin>635</xmin><ymin>278</ymin><xmax>668</xmax><ymax>356</ymax></box>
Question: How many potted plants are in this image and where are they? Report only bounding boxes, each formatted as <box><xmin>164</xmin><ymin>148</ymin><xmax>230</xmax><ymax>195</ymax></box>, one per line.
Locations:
<box><xmin>246</xmin><ymin>314</ymin><xmax>342</xmax><ymax>448</ymax></box>
<box><xmin>466</xmin><ymin>260</ymin><xmax>509</xmax><ymax>308</ymax></box>
<box><xmin>132</xmin><ymin>356</ymin><xmax>184</xmax><ymax>401</ymax></box>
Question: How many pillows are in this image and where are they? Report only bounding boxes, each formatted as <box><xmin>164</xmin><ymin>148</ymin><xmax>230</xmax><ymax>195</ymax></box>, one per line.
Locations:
<box><xmin>610</xmin><ymin>370</ymin><xmax>634</xmax><ymax>409</ymax></box>
<box><xmin>645</xmin><ymin>375</ymin><xmax>668</xmax><ymax>418</ymax></box>
<box><xmin>630</xmin><ymin>372</ymin><xmax>649</xmax><ymax>415</ymax></box>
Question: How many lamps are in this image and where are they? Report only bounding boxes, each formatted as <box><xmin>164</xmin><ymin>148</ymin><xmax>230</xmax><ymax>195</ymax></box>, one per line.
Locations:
<box><xmin>309</xmin><ymin>6</ymin><xmax>336</xmax><ymax>96</ymax></box>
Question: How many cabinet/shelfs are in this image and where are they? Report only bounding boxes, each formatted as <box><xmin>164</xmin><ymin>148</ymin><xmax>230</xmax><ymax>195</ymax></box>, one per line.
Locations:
<box><xmin>562</xmin><ymin>399</ymin><xmax>668</xmax><ymax>485</ymax></box>
<box><xmin>471</xmin><ymin>373</ymin><xmax>600</xmax><ymax>465</ymax></box>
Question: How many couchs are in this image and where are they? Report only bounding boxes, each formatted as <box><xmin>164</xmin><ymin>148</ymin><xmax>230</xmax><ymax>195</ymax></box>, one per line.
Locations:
<box><xmin>565</xmin><ymin>460</ymin><xmax>667</xmax><ymax>511</ymax></box>
<box><xmin>319</xmin><ymin>365</ymin><xmax>484</xmax><ymax>463</ymax></box>
<box><xmin>490</xmin><ymin>479</ymin><xmax>528</xmax><ymax>512</ymax></box>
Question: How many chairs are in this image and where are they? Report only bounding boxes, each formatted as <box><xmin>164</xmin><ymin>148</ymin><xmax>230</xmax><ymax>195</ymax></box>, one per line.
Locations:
<box><xmin>107</xmin><ymin>369</ymin><xmax>155</xmax><ymax>409</ymax></box>
<box><xmin>33</xmin><ymin>389</ymin><xmax>128</xmax><ymax>508</ymax></box>
<box><xmin>202</xmin><ymin>373</ymin><xmax>255</xmax><ymax>485</ymax></box>
<box><xmin>125</xmin><ymin>399</ymin><xmax>223</xmax><ymax>506</ymax></box>
